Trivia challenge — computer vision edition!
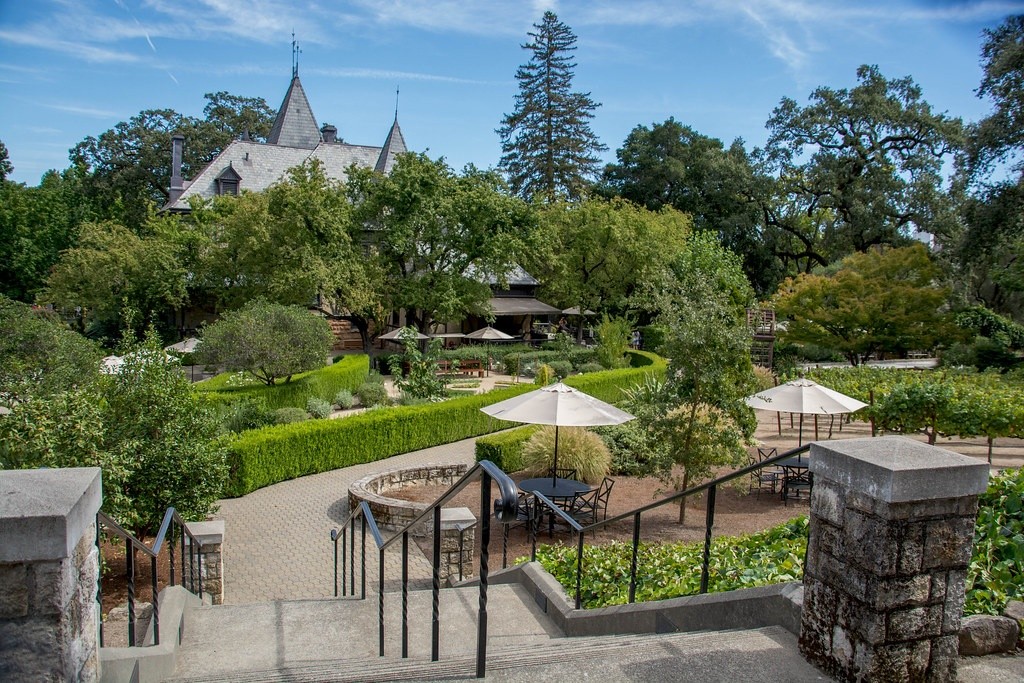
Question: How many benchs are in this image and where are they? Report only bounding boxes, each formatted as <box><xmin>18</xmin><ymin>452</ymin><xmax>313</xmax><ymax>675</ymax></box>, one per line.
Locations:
<box><xmin>403</xmin><ymin>360</ymin><xmax>485</xmax><ymax>378</ymax></box>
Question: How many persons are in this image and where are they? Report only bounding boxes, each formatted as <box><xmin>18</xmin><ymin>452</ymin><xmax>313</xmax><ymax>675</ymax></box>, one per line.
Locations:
<box><xmin>736</xmin><ymin>379</ymin><xmax>870</xmax><ymax>464</ymax></box>
<box><xmin>630</xmin><ymin>329</ymin><xmax>642</xmax><ymax>351</ymax></box>
<box><xmin>559</xmin><ymin>317</ymin><xmax>569</xmax><ymax>333</ymax></box>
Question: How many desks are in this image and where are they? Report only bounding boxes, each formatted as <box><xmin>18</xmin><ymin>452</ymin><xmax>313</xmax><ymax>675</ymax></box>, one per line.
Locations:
<box><xmin>518</xmin><ymin>478</ymin><xmax>591</xmax><ymax>527</ymax></box>
<box><xmin>773</xmin><ymin>457</ymin><xmax>809</xmax><ymax>469</ymax></box>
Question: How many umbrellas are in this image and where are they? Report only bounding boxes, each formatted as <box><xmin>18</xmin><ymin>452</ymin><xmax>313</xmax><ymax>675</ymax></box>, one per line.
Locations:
<box><xmin>377</xmin><ymin>326</ymin><xmax>430</xmax><ymax>341</ymax></box>
<box><xmin>479</xmin><ymin>383</ymin><xmax>637</xmax><ymax>489</ymax></box>
<box><xmin>560</xmin><ymin>305</ymin><xmax>596</xmax><ymax>316</ymax></box>
<box><xmin>463</xmin><ymin>326</ymin><xmax>515</xmax><ymax>378</ymax></box>
<box><xmin>93</xmin><ymin>337</ymin><xmax>206</xmax><ymax>387</ymax></box>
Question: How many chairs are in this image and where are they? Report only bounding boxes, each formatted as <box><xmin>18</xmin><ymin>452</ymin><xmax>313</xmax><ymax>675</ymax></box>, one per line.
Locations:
<box><xmin>747</xmin><ymin>453</ymin><xmax>782</xmax><ymax>501</ymax></box>
<box><xmin>569</xmin><ymin>477</ymin><xmax>615</xmax><ymax>530</ymax></box>
<box><xmin>548</xmin><ymin>468</ymin><xmax>577</xmax><ymax>505</ymax></box>
<box><xmin>517</xmin><ymin>492</ymin><xmax>551</xmax><ymax>543</ymax></box>
<box><xmin>784</xmin><ymin>470</ymin><xmax>813</xmax><ymax>507</ymax></box>
<box><xmin>757</xmin><ymin>448</ymin><xmax>794</xmax><ymax>486</ymax></box>
